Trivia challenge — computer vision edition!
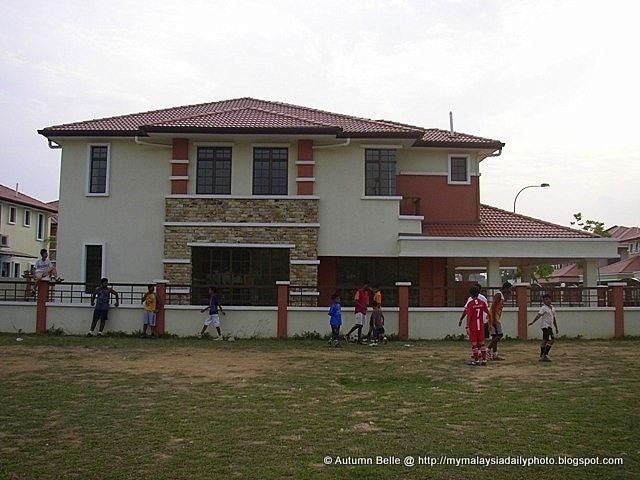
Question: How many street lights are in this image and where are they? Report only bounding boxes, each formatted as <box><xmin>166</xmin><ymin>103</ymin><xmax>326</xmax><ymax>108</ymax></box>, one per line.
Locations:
<box><xmin>511</xmin><ymin>181</ymin><xmax>551</xmax><ymax>215</ymax></box>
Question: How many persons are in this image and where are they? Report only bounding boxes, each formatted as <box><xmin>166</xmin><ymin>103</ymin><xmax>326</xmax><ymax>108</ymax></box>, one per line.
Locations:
<box><xmin>458</xmin><ymin>281</ymin><xmax>512</xmax><ymax>365</ymax></box>
<box><xmin>528</xmin><ymin>294</ymin><xmax>559</xmax><ymax>362</ymax></box>
<box><xmin>33</xmin><ymin>249</ymin><xmax>64</xmax><ymax>291</ymax></box>
<box><xmin>86</xmin><ymin>278</ymin><xmax>119</xmax><ymax>337</ymax></box>
<box><xmin>195</xmin><ymin>287</ymin><xmax>226</xmax><ymax>341</ymax></box>
<box><xmin>327</xmin><ymin>281</ymin><xmax>389</xmax><ymax>347</ymax></box>
<box><xmin>140</xmin><ymin>284</ymin><xmax>162</xmax><ymax>339</ymax></box>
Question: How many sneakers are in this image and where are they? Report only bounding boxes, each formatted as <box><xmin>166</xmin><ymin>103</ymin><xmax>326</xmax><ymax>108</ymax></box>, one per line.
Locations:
<box><xmin>493</xmin><ymin>355</ymin><xmax>505</xmax><ymax>361</ymax></box>
<box><xmin>538</xmin><ymin>354</ymin><xmax>552</xmax><ymax>362</ymax></box>
<box><xmin>343</xmin><ymin>334</ymin><xmax>379</xmax><ymax>347</ymax></box>
<box><xmin>466</xmin><ymin>359</ymin><xmax>488</xmax><ymax>365</ymax></box>
<box><xmin>197</xmin><ymin>333</ymin><xmax>202</xmax><ymax>340</ymax></box>
<box><xmin>213</xmin><ymin>335</ymin><xmax>223</xmax><ymax>341</ymax></box>
<box><xmin>328</xmin><ymin>338</ymin><xmax>341</xmax><ymax>348</ymax></box>
<box><xmin>87</xmin><ymin>332</ymin><xmax>103</xmax><ymax>338</ymax></box>
